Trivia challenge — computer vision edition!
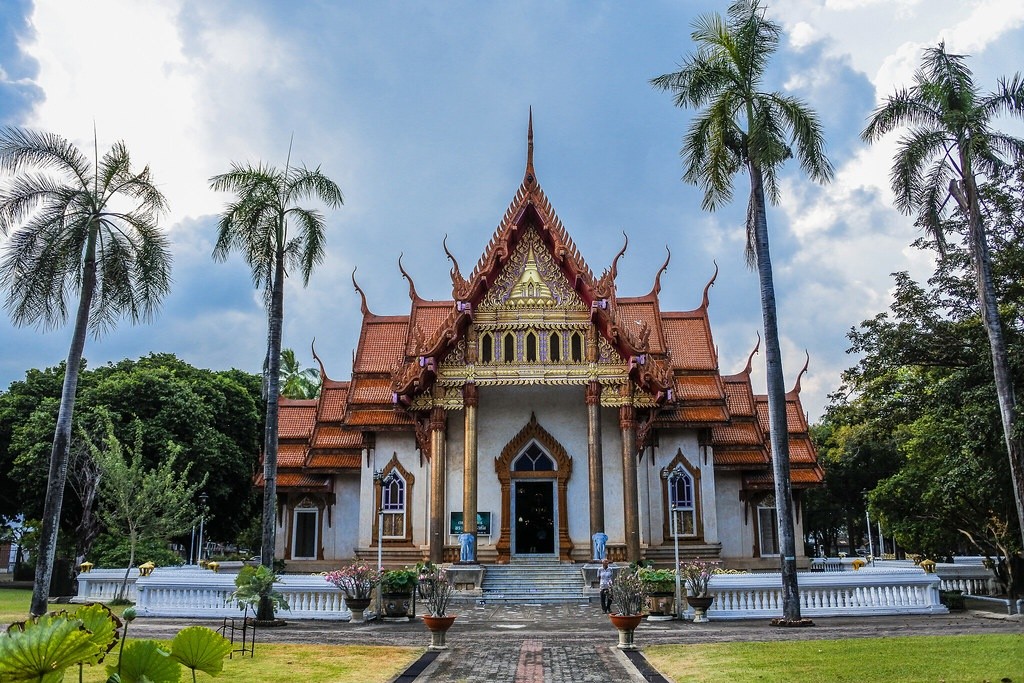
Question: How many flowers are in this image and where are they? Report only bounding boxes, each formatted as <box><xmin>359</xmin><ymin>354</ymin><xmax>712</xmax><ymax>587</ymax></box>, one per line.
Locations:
<box><xmin>320</xmin><ymin>559</ymin><xmax>387</xmax><ymax>599</ymax></box>
<box><xmin>604</xmin><ymin>569</ymin><xmax>655</xmax><ymax>616</ymax></box>
<box><xmin>680</xmin><ymin>556</ymin><xmax>723</xmax><ymax>598</ymax></box>
<box><xmin>417</xmin><ymin>563</ymin><xmax>458</xmax><ymax>616</ymax></box>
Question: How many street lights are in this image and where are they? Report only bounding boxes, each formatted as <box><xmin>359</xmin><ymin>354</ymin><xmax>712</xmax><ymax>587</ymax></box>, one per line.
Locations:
<box><xmin>374</xmin><ymin>468</ymin><xmax>394</xmax><ymax>620</ymax></box>
<box><xmin>663</xmin><ymin>466</ymin><xmax>684</xmax><ymax>619</ymax></box>
<box><xmin>198</xmin><ymin>492</ymin><xmax>209</xmax><ymax>564</ymax></box>
<box><xmin>860</xmin><ymin>486</ymin><xmax>874</xmax><ymax>567</ymax></box>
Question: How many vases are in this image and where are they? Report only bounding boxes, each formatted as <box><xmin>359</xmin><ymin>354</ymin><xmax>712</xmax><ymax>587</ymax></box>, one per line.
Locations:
<box><xmin>686</xmin><ymin>595</ymin><xmax>714</xmax><ymax>623</ymax></box>
<box><xmin>608</xmin><ymin>611</ymin><xmax>643</xmax><ymax>650</ymax></box>
<box><xmin>421</xmin><ymin>613</ymin><xmax>458</xmax><ymax>650</ymax></box>
<box><xmin>344</xmin><ymin>598</ymin><xmax>371</xmax><ymax>624</ymax></box>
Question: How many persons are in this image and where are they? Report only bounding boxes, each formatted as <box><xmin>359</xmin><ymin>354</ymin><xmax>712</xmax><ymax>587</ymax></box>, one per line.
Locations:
<box><xmin>597</xmin><ymin>559</ymin><xmax>614</xmax><ymax>614</ymax></box>
<box><xmin>636</xmin><ymin>556</ymin><xmax>646</xmax><ymax>567</ymax></box>
<box><xmin>946</xmin><ymin>555</ymin><xmax>954</xmax><ymax>562</ymax></box>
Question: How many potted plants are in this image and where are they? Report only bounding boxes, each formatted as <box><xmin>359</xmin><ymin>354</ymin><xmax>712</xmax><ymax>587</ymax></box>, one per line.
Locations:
<box><xmin>376</xmin><ymin>566</ymin><xmax>418</xmax><ymax>617</ymax></box>
<box><xmin>639</xmin><ymin>568</ymin><xmax>677</xmax><ymax>616</ymax></box>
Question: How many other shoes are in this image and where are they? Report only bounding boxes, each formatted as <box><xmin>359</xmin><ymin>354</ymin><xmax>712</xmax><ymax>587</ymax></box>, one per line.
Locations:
<box><xmin>603</xmin><ymin>610</ymin><xmax>606</xmax><ymax>614</ymax></box>
<box><xmin>608</xmin><ymin>608</ymin><xmax>612</xmax><ymax>612</ymax></box>
